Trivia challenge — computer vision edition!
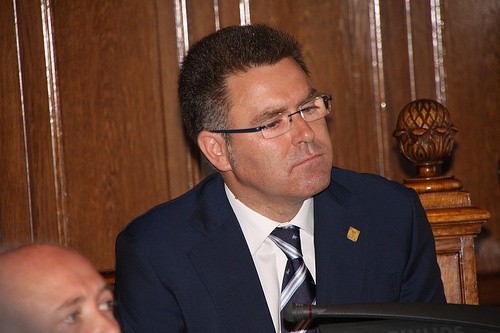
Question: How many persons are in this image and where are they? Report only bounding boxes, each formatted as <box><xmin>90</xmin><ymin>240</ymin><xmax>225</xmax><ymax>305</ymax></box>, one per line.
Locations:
<box><xmin>114</xmin><ymin>23</ymin><xmax>452</xmax><ymax>332</ymax></box>
<box><xmin>0</xmin><ymin>243</ymin><xmax>122</xmax><ymax>332</ymax></box>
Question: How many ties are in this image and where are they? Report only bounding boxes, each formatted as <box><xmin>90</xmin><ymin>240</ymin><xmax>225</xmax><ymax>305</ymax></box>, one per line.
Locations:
<box><xmin>269</xmin><ymin>226</ymin><xmax>315</xmax><ymax>333</ymax></box>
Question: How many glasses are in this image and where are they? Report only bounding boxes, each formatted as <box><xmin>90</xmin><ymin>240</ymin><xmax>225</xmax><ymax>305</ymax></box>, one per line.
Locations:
<box><xmin>207</xmin><ymin>92</ymin><xmax>335</xmax><ymax>137</ymax></box>
